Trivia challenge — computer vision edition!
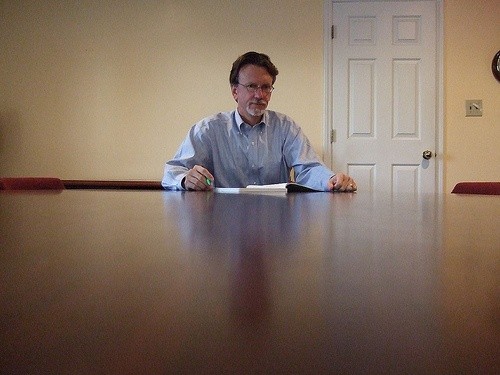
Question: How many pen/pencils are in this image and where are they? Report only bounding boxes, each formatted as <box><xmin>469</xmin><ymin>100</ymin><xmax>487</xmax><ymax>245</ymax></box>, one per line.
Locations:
<box><xmin>206</xmin><ymin>177</ymin><xmax>210</xmax><ymax>185</ymax></box>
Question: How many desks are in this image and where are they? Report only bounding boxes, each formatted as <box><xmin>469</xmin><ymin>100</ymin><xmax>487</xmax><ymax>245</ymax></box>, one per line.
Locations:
<box><xmin>0</xmin><ymin>188</ymin><xmax>500</xmax><ymax>375</ymax></box>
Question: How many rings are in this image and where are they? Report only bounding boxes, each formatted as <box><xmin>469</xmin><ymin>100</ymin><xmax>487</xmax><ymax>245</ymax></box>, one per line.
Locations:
<box><xmin>348</xmin><ymin>183</ymin><xmax>355</xmax><ymax>189</ymax></box>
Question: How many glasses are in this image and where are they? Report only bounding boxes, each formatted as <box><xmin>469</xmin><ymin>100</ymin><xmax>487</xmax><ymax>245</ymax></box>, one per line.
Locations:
<box><xmin>236</xmin><ymin>82</ymin><xmax>274</xmax><ymax>93</ymax></box>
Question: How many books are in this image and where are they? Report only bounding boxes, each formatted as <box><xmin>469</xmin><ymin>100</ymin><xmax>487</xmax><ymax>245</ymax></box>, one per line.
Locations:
<box><xmin>214</xmin><ymin>182</ymin><xmax>325</xmax><ymax>195</ymax></box>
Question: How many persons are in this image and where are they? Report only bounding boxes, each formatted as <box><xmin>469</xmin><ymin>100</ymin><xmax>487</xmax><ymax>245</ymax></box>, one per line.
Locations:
<box><xmin>161</xmin><ymin>51</ymin><xmax>357</xmax><ymax>192</ymax></box>
